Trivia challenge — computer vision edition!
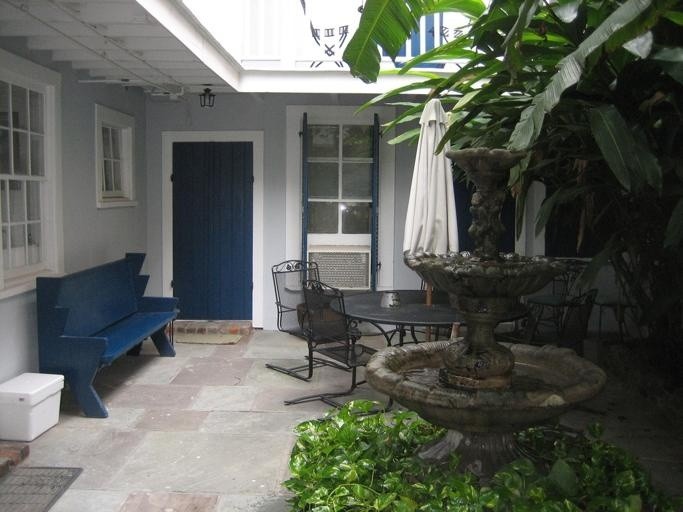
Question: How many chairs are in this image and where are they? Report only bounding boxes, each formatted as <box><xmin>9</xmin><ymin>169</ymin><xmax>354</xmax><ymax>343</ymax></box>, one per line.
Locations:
<box><xmin>265</xmin><ymin>261</ymin><xmax>361</xmax><ymax>382</ymax></box>
<box><xmin>522</xmin><ymin>289</ymin><xmax>597</xmax><ymax>358</ymax></box>
<box><xmin>410</xmin><ymin>278</ymin><xmax>438</xmax><ymax>343</ymax></box>
<box><xmin>528</xmin><ymin>271</ymin><xmax>568</xmax><ymax>342</ymax></box>
<box><xmin>282</xmin><ymin>278</ymin><xmax>396</xmax><ymax>416</ymax></box>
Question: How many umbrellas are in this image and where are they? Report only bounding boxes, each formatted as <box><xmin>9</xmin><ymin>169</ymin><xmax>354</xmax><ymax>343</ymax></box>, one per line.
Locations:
<box><xmin>402</xmin><ymin>97</ymin><xmax>457</xmax><ymax>309</ymax></box>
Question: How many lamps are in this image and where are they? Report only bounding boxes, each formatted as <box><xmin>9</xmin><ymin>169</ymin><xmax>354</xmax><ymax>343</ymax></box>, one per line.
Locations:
<box><xmin>198</xmin><ymin>87</ymin><xmax>215</xmax><ymax>109</ymax></box>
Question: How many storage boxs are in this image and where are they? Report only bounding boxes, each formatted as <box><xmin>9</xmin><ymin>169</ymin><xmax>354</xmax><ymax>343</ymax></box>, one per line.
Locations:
<box><xmin>0</xmin><ymin>373</ymin><xmax>64</xmax><ymax>442</ymax></box>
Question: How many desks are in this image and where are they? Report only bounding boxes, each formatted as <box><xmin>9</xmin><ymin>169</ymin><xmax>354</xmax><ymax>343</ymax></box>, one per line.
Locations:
<box><xmin>330</xmin><ymin>288</ymin><xmax>528</xmax><ymax>348</ymax></box>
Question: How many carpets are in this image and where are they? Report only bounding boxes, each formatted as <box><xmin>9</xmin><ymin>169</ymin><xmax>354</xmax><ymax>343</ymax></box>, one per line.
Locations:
<box><xmin>0</xmin><ymin>467</ymin><xmax>82</xmax><ymax>512</ymax></box>
<box><xmin>175</xmin><ymin>333</ymin><xmax>241</xmax><ymax>344</ymax></box>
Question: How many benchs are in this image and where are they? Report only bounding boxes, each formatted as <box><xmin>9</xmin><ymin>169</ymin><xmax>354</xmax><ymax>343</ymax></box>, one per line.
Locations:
<box><xmin>35</xmin><ymin>253</ymin><xmax>179</xmax><ymax>418</ymax></box>
